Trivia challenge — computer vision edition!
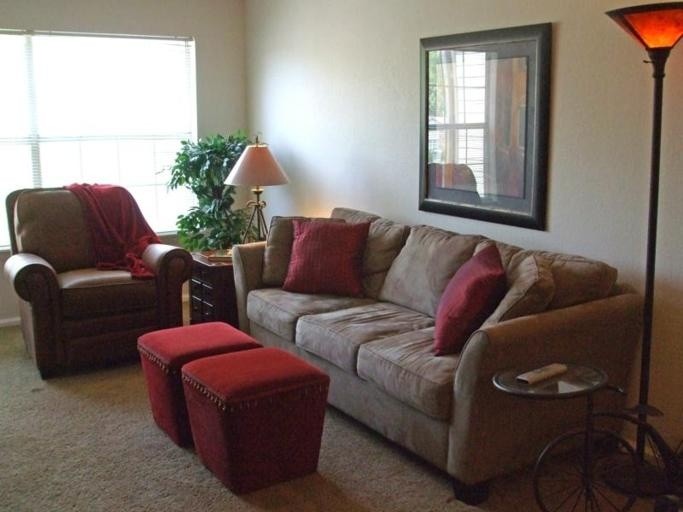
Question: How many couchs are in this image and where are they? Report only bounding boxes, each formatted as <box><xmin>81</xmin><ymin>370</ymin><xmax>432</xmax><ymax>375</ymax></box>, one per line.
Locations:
<box><xmin>230</xmin><ymin>205</ymin><xmax>645</xmax><ymax>508</ymax></box>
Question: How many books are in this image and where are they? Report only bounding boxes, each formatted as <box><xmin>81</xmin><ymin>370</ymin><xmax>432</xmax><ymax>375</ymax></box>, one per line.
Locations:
<box><xmin>208</xmin><ymin>247</ymin><xmax>233</xmax><ymax>262</ymax></box>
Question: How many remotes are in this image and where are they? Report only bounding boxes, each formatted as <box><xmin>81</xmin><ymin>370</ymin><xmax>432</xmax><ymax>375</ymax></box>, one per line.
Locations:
<box><xmin>516</xmin><ymin>362</ymin><xmax>568</xmax><ymax>385</ymax></box>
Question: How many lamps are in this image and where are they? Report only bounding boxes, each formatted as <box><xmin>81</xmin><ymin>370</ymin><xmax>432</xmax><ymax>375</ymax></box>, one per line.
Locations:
<box><xmin>603</xmin><ymin>1</ymin><xmax>682</xmax><ymax>512</ymax></box>
<box><xmin>220</xmin><ymin>133</ymin><xmax>291</xmax><ymax>246</ymax></box>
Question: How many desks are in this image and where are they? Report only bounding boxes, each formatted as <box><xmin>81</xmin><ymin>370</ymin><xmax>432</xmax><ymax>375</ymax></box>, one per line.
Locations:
<box><xmin>488</xmin><ymin>357</ymin><xmax>630</xmax><ymax>495</ymax></box>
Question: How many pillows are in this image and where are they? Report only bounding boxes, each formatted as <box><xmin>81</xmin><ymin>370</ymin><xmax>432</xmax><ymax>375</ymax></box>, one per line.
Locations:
<box><xmin>478</xmin><ymin>252</ymin><xmax>557</xmax><ymax>331</ymax></box>
<box><xmin>428</xmin><ymin>242</ymin><xmax>510</xmax><ymax>358</ymax></box>
<box><xmin>261</xmin><ymin>214</ymin><xmax>346</xmax><ymax>287</ymax></box>
<box><xmin>282</xmin><ymin>217</ymin><xmax>372</xmax><ymax>301</ymax></box>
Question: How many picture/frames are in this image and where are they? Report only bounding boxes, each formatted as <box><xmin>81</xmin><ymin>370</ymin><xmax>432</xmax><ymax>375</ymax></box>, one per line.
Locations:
<box><xmin>416</xmin><ymin>21</ymin><xmax>554</xmax><ymax>233</ymax></box>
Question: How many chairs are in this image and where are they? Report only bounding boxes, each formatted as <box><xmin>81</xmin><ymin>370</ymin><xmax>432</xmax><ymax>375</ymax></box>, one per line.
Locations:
<box><xmin>0</xmin><ymin>184</ymin><xmax>195</xmax><ymax>383</ymax></box>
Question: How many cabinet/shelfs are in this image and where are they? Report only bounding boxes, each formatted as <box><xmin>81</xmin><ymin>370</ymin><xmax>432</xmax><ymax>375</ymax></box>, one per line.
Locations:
<box><xmin>185</xmin><ymin>244</ymin><xmax>241</xmax><ymax>332</ymax></box>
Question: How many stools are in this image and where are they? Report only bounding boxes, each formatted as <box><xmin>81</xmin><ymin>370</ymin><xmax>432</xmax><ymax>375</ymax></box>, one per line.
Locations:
<box><xmin>177</xmin><ymin>343</ymin><xmax>331</xmax><ymax>497</ymax></box>
<box><xmin>135</xmin><ymin>320</ymin><xmax>264</xmax><ymax>451</ymax></box>
